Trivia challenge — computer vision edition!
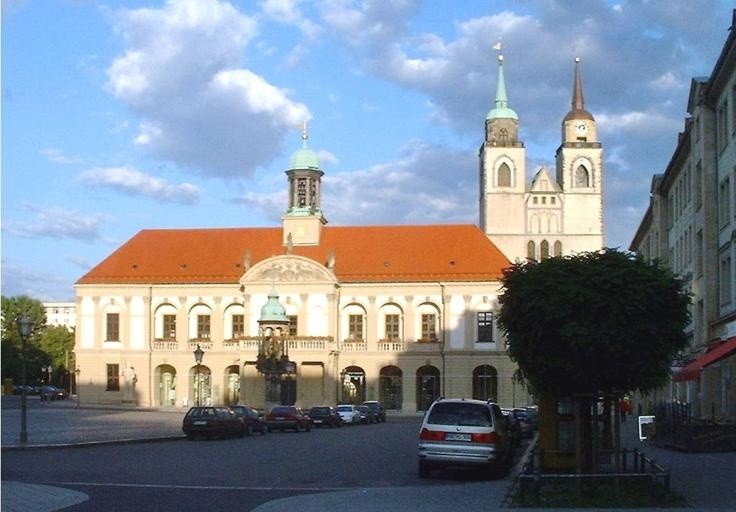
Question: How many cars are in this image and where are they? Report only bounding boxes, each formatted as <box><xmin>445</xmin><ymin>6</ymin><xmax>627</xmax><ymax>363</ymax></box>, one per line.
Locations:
<box><xmin>179</xmin><ymin>396</ymin><xmax>386</xmax><ymax>443</ymax></box>
<box><xmin>10</xmin><ymin>385</ymin><xmax>69</xmax><ymax>400</ymax></box>
<box><xmin>589</xmin><ymin>396</ymin><xmax>605</xmax><ymax>418</ymax></box>
<box><xmin>414</xmin><ymin>394</ymin><xmax>539</xmax><ymax>479</ymax></box>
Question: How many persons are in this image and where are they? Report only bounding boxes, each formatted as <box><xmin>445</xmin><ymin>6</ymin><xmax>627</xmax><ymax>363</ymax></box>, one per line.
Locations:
<box><xmin>619</xmin><ymin>398</ymin><xmax>629</xmax><ymax>422</ymax></box>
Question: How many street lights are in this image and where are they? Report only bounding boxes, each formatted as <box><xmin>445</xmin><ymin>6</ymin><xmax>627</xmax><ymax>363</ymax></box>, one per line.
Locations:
<box><xmin>15</xmin><ymin>313</ymin><xmax>37</xmax><ymax>441</ymax></box>
<box><xmin>194</xmin><ymin>343</ymin><xmax>205</xmax><ymax>405</ymax></box>
